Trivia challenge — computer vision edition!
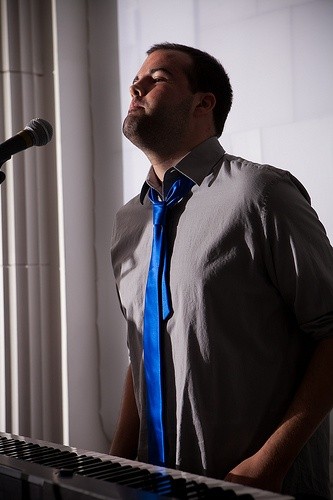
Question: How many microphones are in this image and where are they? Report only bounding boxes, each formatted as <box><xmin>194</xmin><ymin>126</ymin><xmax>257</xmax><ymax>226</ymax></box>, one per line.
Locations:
<box><xmin>0</xmin><ymin>117</ymin><xmax>53</xmax><ymax>169</ymax></box>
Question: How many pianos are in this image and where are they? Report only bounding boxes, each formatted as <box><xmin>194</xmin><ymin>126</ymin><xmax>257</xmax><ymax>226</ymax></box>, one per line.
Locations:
<box><xmin>0</xmin><ymin>432</ymin><xmax>296</xmax><ymax>500</ymax></box>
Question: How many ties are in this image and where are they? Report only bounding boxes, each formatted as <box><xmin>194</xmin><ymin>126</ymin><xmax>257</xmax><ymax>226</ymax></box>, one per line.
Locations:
<box><xmin>143</xmin><ymin>181</ymin><xmax>181</xmax><ymax>464</ymax></box>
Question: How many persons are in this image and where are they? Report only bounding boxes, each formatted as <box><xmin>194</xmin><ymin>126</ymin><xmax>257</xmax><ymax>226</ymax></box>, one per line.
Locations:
<box><xmin>108</xmin><ymin>43</ymin><xmax>333</xmax><ymax>500</ymax></box>
